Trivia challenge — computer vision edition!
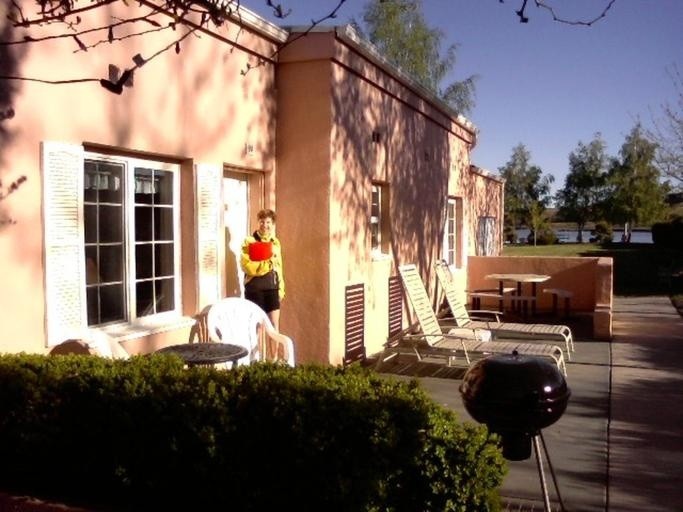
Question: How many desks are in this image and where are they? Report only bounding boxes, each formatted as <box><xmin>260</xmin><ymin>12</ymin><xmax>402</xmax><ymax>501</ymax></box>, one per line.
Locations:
<box><xmin>153</xmin><ymin>342</ymin><xmax>249</xmax><ymax>379</ymax></box>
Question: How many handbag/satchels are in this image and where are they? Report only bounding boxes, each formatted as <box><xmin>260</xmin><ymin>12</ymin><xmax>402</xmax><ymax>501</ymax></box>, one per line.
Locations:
<box><xmin>252</xmin><ymin>261</ymin><xmax>278</xmax><ymax>290</ymax></box>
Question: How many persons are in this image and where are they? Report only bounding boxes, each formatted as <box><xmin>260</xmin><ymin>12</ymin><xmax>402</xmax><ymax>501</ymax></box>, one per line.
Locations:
<box><xmin>240</xmin><ymin>210</ymin><xmax>285</xmax><ymax>333</ymax></box>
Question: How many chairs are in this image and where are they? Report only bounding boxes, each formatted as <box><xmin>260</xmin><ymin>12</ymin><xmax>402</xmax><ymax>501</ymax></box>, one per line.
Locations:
<box><xmin>206</xmin><ymin>294</ymin><xmax>296</xmax><ymax>371</ymax></box>
<box><xmin>375</xmin><ymin>258</ymin><xmax>575</xmax><ymax>377</ymax></box>
